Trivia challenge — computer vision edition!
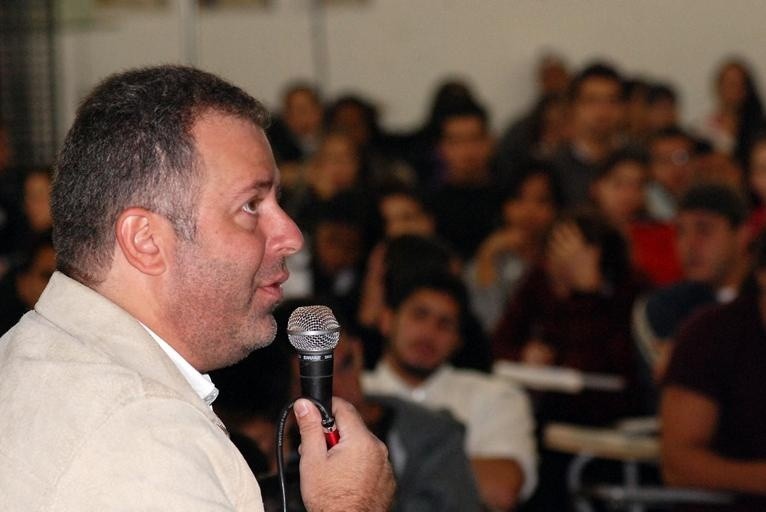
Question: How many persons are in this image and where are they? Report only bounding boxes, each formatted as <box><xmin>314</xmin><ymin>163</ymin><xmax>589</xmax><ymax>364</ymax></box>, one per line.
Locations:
<box><xmin>210</xmin><ymin>55</ymin><xmax>765</xmax><ymax>511</ymax></box>
<box><xmin>0</xmin><ymin>64</ymin><xmax>398</xmax><ymax>512</ymax></box>
<box><xmin>1</xmin><ymin>147</ymin><xmax>58</xmax><ymax>339</ymax></box>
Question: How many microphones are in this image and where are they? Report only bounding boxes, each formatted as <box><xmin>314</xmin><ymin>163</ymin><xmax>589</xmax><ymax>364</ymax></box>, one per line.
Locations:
<box><xmin>286</xmin><ymin>303</ymin><xmax>341</xmax><ymax>449</ymax></box>
